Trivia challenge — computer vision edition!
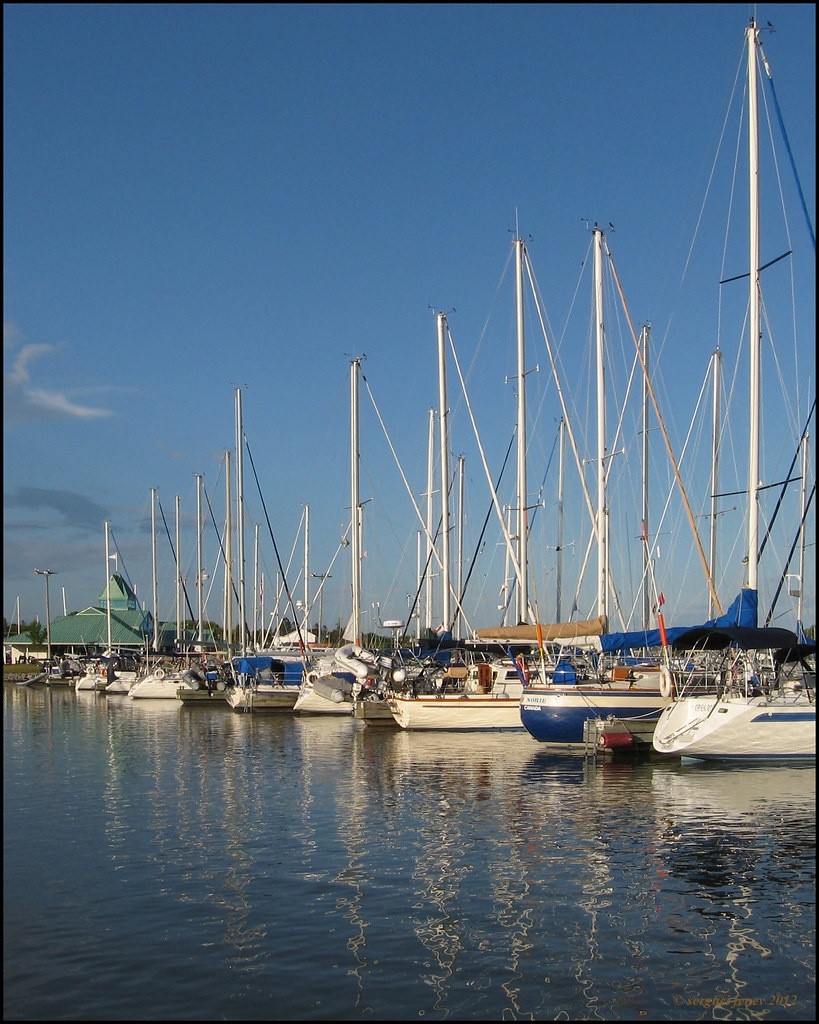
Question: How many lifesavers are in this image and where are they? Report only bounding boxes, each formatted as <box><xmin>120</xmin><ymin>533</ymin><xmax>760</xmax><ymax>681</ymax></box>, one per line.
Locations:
<box><xmin>659</xmin><ymin>666</ymin><xmax>672</xmax><ymax>698</ymax></box>
<box><xmin>87</xmin><ymin>666</ymin><xmax>96</xmax><ymax>674</ymax></box>
<box><xmin>307</xmin><ymin>671</ymin><xmax>320</xmax><ymax>687</ymax></box>
<box><xmin>103</xmin><ymin>668</ymin><xmax>109</xmax><ymax>677</ymax></box>
<box><xmin>517</xmin><ymin>660</ymin><xmax>530</xmax><ymax>683</ymax></box>
<box><xmin>155</xmin><ymin>668</ymin><xmax>166</xmax><ymax>680</ymax></box>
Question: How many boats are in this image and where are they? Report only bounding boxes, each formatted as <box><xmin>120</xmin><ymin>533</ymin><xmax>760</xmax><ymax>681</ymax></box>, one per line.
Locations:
<box><xmin>33</xmin><ymin>3</ymin><xmax>816</xmax><ymax>758</ymax></box>
